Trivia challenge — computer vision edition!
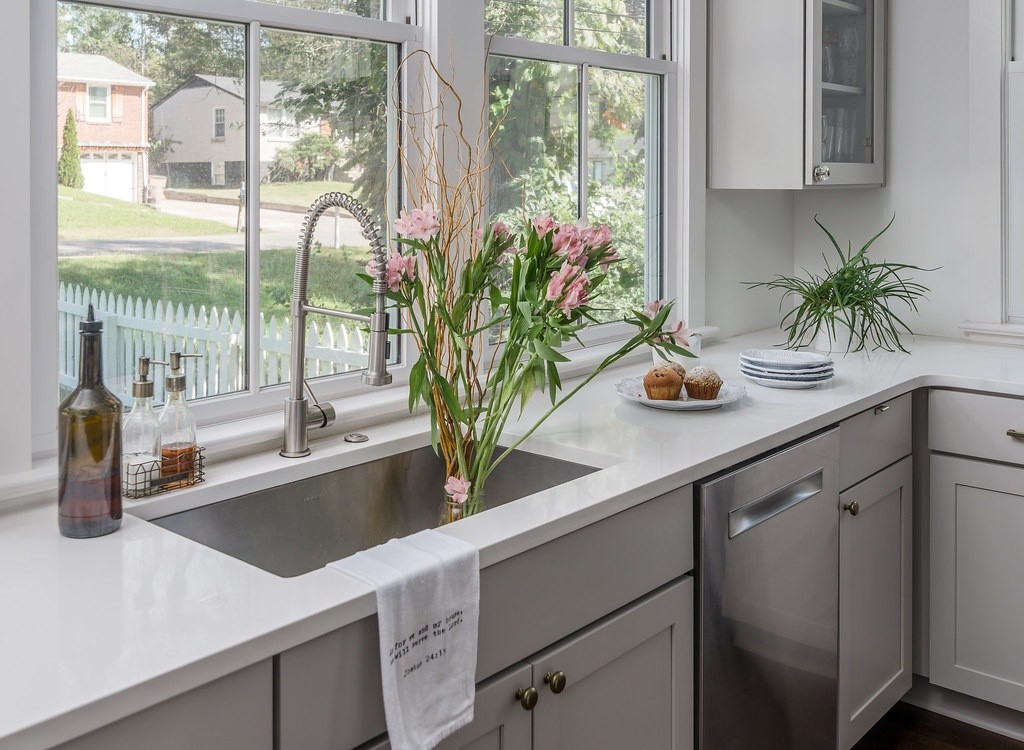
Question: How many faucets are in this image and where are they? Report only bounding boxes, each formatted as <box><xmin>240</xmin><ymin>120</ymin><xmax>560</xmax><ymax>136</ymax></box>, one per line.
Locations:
<box><xmin>276</xmin><ymin>192</ymin><xmax>399</xmax><ymax>460</ymax></box>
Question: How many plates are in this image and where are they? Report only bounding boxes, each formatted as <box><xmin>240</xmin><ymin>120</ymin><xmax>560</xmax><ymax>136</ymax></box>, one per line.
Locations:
<box><xmin>615</xmin><ymin>373</ymin><xmax>749</xmax><ymax>412</ymax></box>
<box><xmin>735</xmin><ymin>348</ymin><xmax>836</xmax><ymax>390</ymax></box>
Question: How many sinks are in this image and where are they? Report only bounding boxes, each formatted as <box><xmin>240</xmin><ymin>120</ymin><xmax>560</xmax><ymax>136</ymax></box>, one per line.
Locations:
<box><xmin>118</xmin><ymin>426</ymin><xmax>628</xmax><ymax>580</ymax></box>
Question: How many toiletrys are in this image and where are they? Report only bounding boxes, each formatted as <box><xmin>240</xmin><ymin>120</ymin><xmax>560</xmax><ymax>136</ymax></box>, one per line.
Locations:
<box><xmin>156</xmin><ymin>349</ymin><xmax>208</xmax><ymax>490</ymax></box>
<box><xmin>114</xmin><ymin>356</ymin><xmax>173</xmax><ymax>499</ymax></box>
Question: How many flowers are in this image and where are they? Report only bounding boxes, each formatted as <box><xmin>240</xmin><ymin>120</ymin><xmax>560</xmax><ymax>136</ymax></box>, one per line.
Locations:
<box><xmin>354</xmin><ymin>205</ymin><xmax>706</xmax><ymax>525</ymax></box>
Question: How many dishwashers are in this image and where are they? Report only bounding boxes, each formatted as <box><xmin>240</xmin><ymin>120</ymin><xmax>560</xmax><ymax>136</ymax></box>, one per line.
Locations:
<box><xmin>685</xmin><ymin>428</ymin><xmax>841</xmax><ymax>750</ymax></box>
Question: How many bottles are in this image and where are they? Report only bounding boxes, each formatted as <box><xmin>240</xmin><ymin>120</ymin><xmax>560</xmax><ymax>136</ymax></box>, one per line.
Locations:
<box><xmin>156</xmin><ymin>349</ymin><xmax>200</xmax><ymax>489</ymax></box>
<box><xmin>120</xmin><ymin>354</ymin><xmax>164</xmax><ymax>491</ymax></box>
<box><xmin>57</xmin><ymin>305</ymin><xmax>123</xmax><ymax>540</ymax></box>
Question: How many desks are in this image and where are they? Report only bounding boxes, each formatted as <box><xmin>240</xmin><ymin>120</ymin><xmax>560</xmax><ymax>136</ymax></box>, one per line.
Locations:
<box><xmin>837</xmin><ymin>395</ymin><xmax>915</xmax><ymax>750</ymax></box>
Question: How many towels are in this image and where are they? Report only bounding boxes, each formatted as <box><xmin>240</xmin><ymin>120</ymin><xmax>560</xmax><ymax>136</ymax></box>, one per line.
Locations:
<box><xmin>323</xmin><ymin>527</ymin><xmax>483</xmax><ymax>750</ymax></box>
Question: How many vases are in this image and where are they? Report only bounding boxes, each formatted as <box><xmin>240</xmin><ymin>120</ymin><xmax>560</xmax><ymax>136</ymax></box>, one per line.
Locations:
<box><xmin>438</xmin><ymin>487</ymin><xmax>486</xmax><ymax>527</ymax></box>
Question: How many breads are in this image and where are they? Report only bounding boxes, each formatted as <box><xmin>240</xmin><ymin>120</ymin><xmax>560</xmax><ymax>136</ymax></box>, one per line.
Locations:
<box><xmin>643</xmin><ymin>360</ymin><xmax>723</xmax><ymax>401</ymax></box>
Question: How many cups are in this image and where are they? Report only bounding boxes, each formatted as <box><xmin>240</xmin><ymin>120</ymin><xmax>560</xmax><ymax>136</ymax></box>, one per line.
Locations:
<box><xmin>651</xmin><ymin>332</ymin><xmax>704</xmax><ymax>372</ymax></box>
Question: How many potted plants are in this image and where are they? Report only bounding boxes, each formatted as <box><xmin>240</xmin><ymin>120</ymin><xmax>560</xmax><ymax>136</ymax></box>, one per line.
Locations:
<box><xmin>737</xmin><ymin>211</ymin><xmax>944</xmax><ymax>363</ymax></box>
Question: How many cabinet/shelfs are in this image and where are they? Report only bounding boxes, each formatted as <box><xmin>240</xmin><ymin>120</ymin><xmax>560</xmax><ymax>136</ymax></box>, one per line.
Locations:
<box><xmin>709</xmin><ymin>2</ymin><xmax>892</xmax><ymax>193</ymax></box>
<box><xmin>366</xmin><ymin>574</ymin><xmax>697</xmax><ymax>749</ymax></box>
<box><xmin>922</xmin><ymin>386</ymin><xmax>1023</xmax><ymax>746</ymax></box>
